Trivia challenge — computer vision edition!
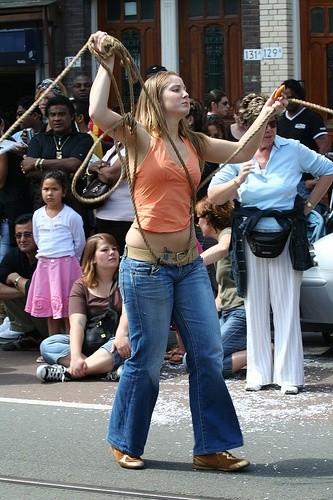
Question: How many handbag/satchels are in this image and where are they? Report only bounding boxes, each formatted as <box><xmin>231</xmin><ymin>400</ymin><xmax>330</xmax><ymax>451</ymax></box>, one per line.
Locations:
<box><xmin>73</xmin><ymin>144</ymin><xmax>124</xmax><ymax>209</ymax></box>
<box><xmin>83</xmin><ymin>309</ymin><xmax>118</xmax><ymax>351</ymax></box>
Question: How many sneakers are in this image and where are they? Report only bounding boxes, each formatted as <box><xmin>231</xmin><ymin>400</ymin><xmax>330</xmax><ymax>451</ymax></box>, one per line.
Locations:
<box><xmin>102</xmin><ymin>364</ymin><xmax>124</xmax><ymax>381</ymax></box>
<box><xmin>37</xmin><ymin>363</ymin><xmax>71</xmax><ymax>382</ymax></box>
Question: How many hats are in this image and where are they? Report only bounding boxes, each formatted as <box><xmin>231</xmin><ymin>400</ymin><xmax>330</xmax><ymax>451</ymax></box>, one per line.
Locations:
<box><xmin>145</xmin><ymin>65</ymin><xmax>166</xmax><ymax>76</ymax></box>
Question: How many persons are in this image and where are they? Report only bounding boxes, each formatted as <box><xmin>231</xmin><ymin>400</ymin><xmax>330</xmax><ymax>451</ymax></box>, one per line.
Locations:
<box><xmin>160</xmin><ymin>195</ymin><xmax>279</xmax><ymax>378</ymax></box>
<box><xmin>206</xmin><ymin>93</ymin><xmax>333</xmax><ymax>394</ymax></box>
<box><xmin>87</xmin><ymin>30</ymin><xmax>287</xmax><ymax>473</ymax></box>
<box><xmin>35</xmin><ymin>232</ymin><xmax>130</xmax><ymax>383</ymax></box>
<box><xmin>0</xmin><ymin>72</ymin><xmax>117</xmax><ymax>351</ymax></box>
<box><xmin>31</xmin><ymin>171</ymin><xmax>85</xmax><ymax>363</ymax></box>
<box><xmin>85</xmin><ymin>65</ymin><xmax>333</xmax><ymax>256</ymax></box>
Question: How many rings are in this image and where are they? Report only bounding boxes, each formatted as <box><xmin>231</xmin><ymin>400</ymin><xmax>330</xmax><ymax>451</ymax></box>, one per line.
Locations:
<box><xmin>21</xmin><ymin>169</ymin><xmax>23</xmax><ymax>171</ymax></box>
<box><xmin>23</xmin><ymin>155</ymin><xmax>27</xmax><ymax>159</ymax></box>
<box><xmin>20</xmin><ymin>165</ymin><xmax>22</xmax><ymax>168</ymax></box>
<box><xmin>22</xmin><ymin>171</ymin><xmax>25</xmax><ymax>173</ymax></box>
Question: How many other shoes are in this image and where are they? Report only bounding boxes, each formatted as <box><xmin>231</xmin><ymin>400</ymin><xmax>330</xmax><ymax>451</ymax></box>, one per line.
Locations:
<box><xmin>165</xmin><ymin>347</ymin><xmax>184</xmax><ymax>364</ymax></box>
<box><xmin>0</xmin><ymin>317</ymin><xmax>22</xmax><ymax>339</ymax></box>
<box><xmin>283</xmin><ymin>386</ymin><xmax>299</xmax><ymax>394</ymax></box>
<box><xmin>110</xmin><ymin>447</ymin><xmax>144</xmax><ymax>468</ymax></box>
<box><xmin>3</xmin><ymin>334</ymin><xmax>42</xmax><ymax>351</ymax></box>
<box><xmin>36</xmin><ymin>355</ymin><xmax>44</xmax><ymax>362</ymax></box>
<box><xmin>245</xmin><ymin>384</ymin><xmax>261</xmax><ymax>390</ymax></box>
<box><xmin>194</xmin><ymin>450</ymin><xmax>251</xmax><ymax>472</ymax></box>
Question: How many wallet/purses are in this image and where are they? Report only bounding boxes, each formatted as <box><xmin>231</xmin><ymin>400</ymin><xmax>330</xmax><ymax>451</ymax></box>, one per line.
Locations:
<box><xmin>245</xmin><ymin>229</ymin><xmax>288</xmax><ymax>258</ymax></box>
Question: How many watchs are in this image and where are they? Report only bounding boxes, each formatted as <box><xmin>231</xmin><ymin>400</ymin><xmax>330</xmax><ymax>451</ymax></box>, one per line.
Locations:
<box><xmin>13</xmin><ymin>276</ymin><xmax>22</xmax><ymax>289</ymax></box>
<box><xmin>304</xmin><ymin>200</ymin><xmax>314</xmax><ymax>209</ymax></box>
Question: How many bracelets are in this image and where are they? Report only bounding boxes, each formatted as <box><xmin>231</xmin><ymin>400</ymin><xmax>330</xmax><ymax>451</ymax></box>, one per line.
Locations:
<box><xmin>233</xmin><ymin>177</ymin><xmax>239</xmax><ymax>188</ymax></box>
<box><xmin>34</xmin><ymin>158</ymin><xmax>39</xmax><ymax>172</ymax></box>
<box><xmin>38</xmin><ymin>159</ymin><xmax>44</xmax><ymax>172</ymax></box>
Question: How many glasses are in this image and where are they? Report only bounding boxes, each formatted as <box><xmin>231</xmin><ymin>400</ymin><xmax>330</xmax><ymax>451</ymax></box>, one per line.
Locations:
<box><xmin>16</xmin><ymin>112</ymin><xmax>24</xmax><ymax>116</ymax></box>
<box><xmin>194</xmin><ymin>217</ymin><xmax>200</xmax><ymax>223</ymax></box>
<box><xmin>37</xmin><ymin>79</ymin><xmax>60</xmax><ymax>95</ymax></box>
<box><xmin>221</xmin><ymin>100</ymin><xmax>228</xmax><ymax>106</ymax></box>
<box><xmin>14</xmin><ymin>232</ymin><xmax>33</xmax><ymax>238</ymax></box>
<box><xmin>267</xmin><ymin>120</ymin><xmax>278</xmax><ymax>128</ymax></box>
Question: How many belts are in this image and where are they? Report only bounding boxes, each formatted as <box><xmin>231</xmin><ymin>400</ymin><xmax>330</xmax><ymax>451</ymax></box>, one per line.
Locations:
<box><xmin>127</xmin><ymin>246</ymin><xmax>198</xmax><ymax>266</ymax></box>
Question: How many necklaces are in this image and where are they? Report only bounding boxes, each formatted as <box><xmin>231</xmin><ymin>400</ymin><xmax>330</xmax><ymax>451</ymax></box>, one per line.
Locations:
<box><xmin>257</xmin><ymin>159</ymin><xmax>267</xmax><ymax>163</ymax></box>
<box><xmin>51</xmin><ymin>135</ymin><xmax>71</xmax><ymax>160</ymax></box>
